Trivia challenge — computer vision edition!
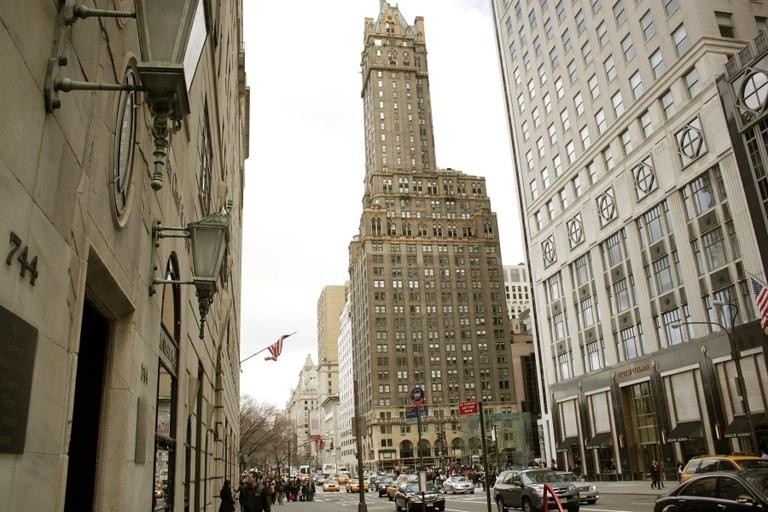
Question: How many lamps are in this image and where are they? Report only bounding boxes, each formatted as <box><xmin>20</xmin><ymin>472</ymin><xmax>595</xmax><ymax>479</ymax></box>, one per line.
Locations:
<box><xmin>148</xmin><ymin>204</ymin><xmax>231</xmax><ymax>339</ymax></box>
<box><xmin>43</xmin><ymin>0</ymin><xmax>211</xmax><ymax>192</ymax></box>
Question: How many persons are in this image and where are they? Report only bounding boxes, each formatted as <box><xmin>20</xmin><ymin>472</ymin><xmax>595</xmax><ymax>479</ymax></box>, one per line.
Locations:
<box><xmin>262</xmin><ymin>474</ymin><xmax>315</xmax><ymax>505</ymax></box>
<box><xmin>761</xmin><ymin>448</ymin><xmax>768</xmax><ymax>458</ymax></box>
<box><xmin>218</xmin><ymin>479</ymin><xmax>235</xmax><ymax>512</ymax></box>
<box><xmin>233</xmin><ymin>481</ymin><xmax>246</xmax><ymax>512</ymax></box>
<box><xmin>648</xmin><ymin>457</ymin><xmax>685</xmax><ymax>490</ymax></box>
<box><xmin>424</xmin><ymin>457</ymin><xmax>583</xmax><ymax>495</ymax></box>
<box><xmin>239</xmin><ymin>470</ymin><xmax>271</xmax><ymax>512</ymax></box>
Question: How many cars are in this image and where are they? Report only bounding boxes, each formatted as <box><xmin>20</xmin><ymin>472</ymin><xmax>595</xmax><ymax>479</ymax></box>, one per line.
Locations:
<box><xmin>654</xmin><ymin>455</ymin><xmax>768</xmax><ymax>512</ymax></box>
<box><xmin>337</xmin><ymin>467</ymin><xmax>348</xmax><ymax>473</ymax></box>
<box><xmin>493</xmin><ymin>468</ymin><xmax>599</xmax><ymax>512</ymax></box>
<box><xmin>379</xmin><ymin>473</ymin><xmax>445</xmax><ymax>512</ymax></box>
<box><xmin>442</xmin><ymin>476</ymin><xmax>475</xmax><ymax>494</ymax></box>
<box><xmin>300</xmin><ymin>465</ymin><xmax>310</xmax><ymax>475</ymax></box>
<box><xmin>313</xmin><ymin>473</ymin><xmax>369</xmax><ymax>493</ymax></box>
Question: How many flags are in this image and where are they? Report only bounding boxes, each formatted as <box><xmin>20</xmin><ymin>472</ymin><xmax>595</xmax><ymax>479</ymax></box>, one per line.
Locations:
<box><xmin>264</xmin><ymin>334</ymin><xmax>292</xmax><ymax>361</ymax></box>
<box><xmin>744</xmin><ymin>269</ymin><xmax>768</xmax><ymax>336</ymax></box>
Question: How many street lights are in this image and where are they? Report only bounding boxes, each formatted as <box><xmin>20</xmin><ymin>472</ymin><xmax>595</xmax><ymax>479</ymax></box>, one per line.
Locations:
<box><xmin>671</xmin><ymin>301</ymin><xmax>764</xmax><ymax>456</ymax></box>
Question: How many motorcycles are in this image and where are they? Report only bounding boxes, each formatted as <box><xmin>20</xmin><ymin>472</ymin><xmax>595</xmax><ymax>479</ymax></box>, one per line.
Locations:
<box><xmin>370</xmin><ymin>477</ymin><xmax>378</xmax><ymax>491</ymax></box>
